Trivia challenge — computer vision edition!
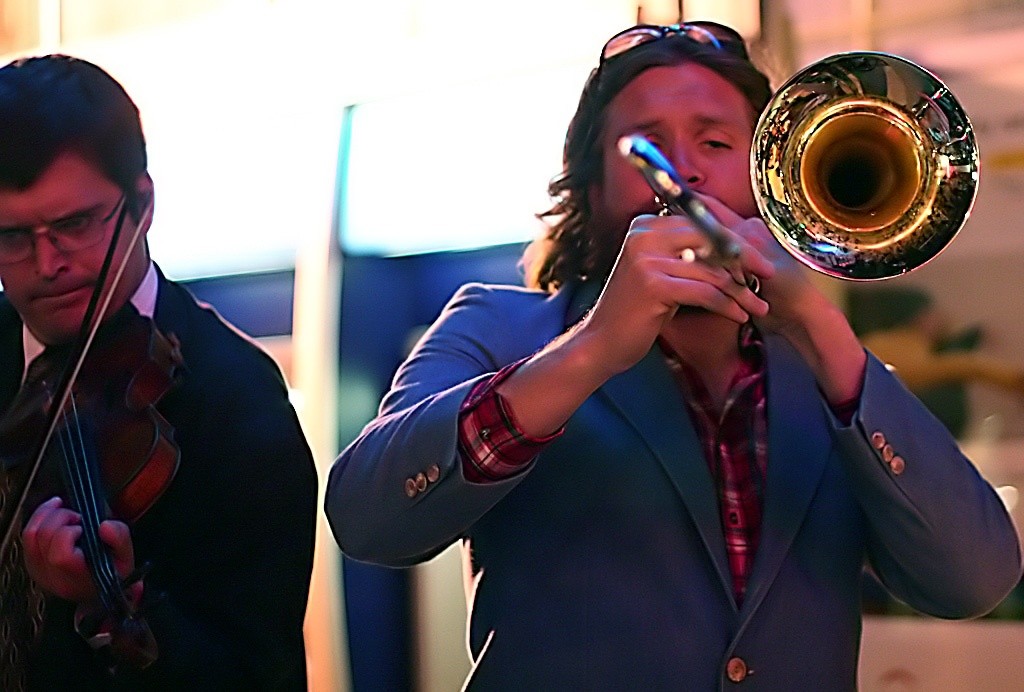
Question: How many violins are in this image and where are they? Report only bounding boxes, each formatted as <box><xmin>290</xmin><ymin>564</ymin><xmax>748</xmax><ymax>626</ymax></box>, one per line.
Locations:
<box><xmin>9</xmin><ymin>300</ymin><xmax>185</xmax><ymax>669</ymax></box>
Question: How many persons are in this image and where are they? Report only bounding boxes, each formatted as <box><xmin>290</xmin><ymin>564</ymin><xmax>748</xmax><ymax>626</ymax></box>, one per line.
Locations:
<box><xmin>323</xmin><ymin>20</ymin><xmax>1024</xmax><ymax>692</ymax></box>
<box><xmin>0</xmin><ymin>55</ymin><xmax>317</xmax><ymax>692</ymax></box>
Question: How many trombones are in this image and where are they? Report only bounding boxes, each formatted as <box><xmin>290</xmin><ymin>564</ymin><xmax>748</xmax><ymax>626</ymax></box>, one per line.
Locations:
<box><xmin>616</xmin><ymin>51</ymin><xmax>982</xmax><ymax>292</ymax></box>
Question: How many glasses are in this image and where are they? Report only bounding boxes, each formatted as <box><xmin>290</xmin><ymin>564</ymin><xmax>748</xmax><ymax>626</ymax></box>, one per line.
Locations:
<box><xmin>0</xmin><ymin>182</ymin><xmax>131</xmax><ymax>266</ymax></box>
<box><xmin>592</xmin><ymin>21</ymin><xmax>747</xmax><ymax>80</ymax></box>
